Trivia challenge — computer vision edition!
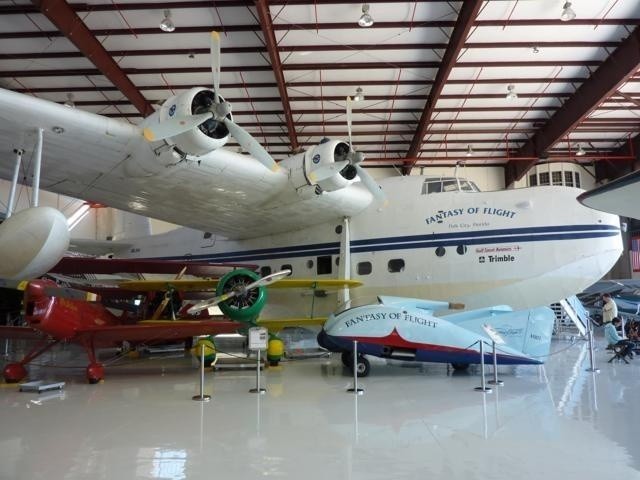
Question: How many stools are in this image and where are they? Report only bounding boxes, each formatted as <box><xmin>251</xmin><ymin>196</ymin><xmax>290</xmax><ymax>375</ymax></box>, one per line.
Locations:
<box><xmin>606</xmin><ymin>345</ymin><xmax>629</xmax><ymax>364</ymax></box>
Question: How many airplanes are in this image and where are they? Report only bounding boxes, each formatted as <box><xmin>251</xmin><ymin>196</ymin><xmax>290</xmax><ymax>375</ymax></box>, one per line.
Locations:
<box><xmin>317</xmin><ymin>296</ymin><xmax>556</xmax><ymax>378</ymax></box>
<box><xmin>1</xmin><ymin>31</ymin><xmax>630</xmax><ymax>367</ymax></box>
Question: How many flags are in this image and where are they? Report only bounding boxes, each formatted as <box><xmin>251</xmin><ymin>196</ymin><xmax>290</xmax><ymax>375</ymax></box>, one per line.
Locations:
<box><xmin>631</xmin><ymin>236</ymin><xmax>640</xmax><ymax>272</ymax></box>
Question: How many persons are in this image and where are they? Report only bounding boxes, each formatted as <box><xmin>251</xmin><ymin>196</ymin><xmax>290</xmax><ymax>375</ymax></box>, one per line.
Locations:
<box><xmin>602</xmin><ymin>294</ymin><xmax>617</xmax><ymax>349</ymax></box>
<box><xmin>604</xmin><ymin>316</ymin><xmax>635</xmax><ymax>359</ymax></box>
<box><xmin>624</xmin><ymin>318</ymin><xmax>640</xmax><ymax>355</ymax></box>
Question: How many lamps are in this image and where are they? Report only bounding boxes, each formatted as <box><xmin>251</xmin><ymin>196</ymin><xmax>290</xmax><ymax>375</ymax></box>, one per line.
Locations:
<box><xmin>466</xmin><ymin>145</ymin><xmax>475</xmax><ymax>156</ymax></box>
<box><xmin>358</xmin><ymin>4</ymin><xmax>374</xmax><ymax>27</ymax></box>
<box><xmin>354</xmin><ymin>87</ymin><xmax>364</xmax><ymax>101</ymax></box>
<box><xmin>64</xmin><ymin>92</ymin><xmax>75</xmax><ymax>108</ymax></box>
<box><xmin>506</xmin><ymin>85</ymin><xmax>518</xmax><ymax>99</ymax></box>
<box><xmin>575</xmin><ymin>143</ymin><xmax>586</xmax><ymax>156</ymax></box>
<box><xmin>560</xmin><ymin>2</ymin><xmax>577</xmax><ymax>21</ymax></box>
<box><xmin>160</xmin><ymin>9</ymin><xmax>176</xmax><ymax>32</ymax></box>
